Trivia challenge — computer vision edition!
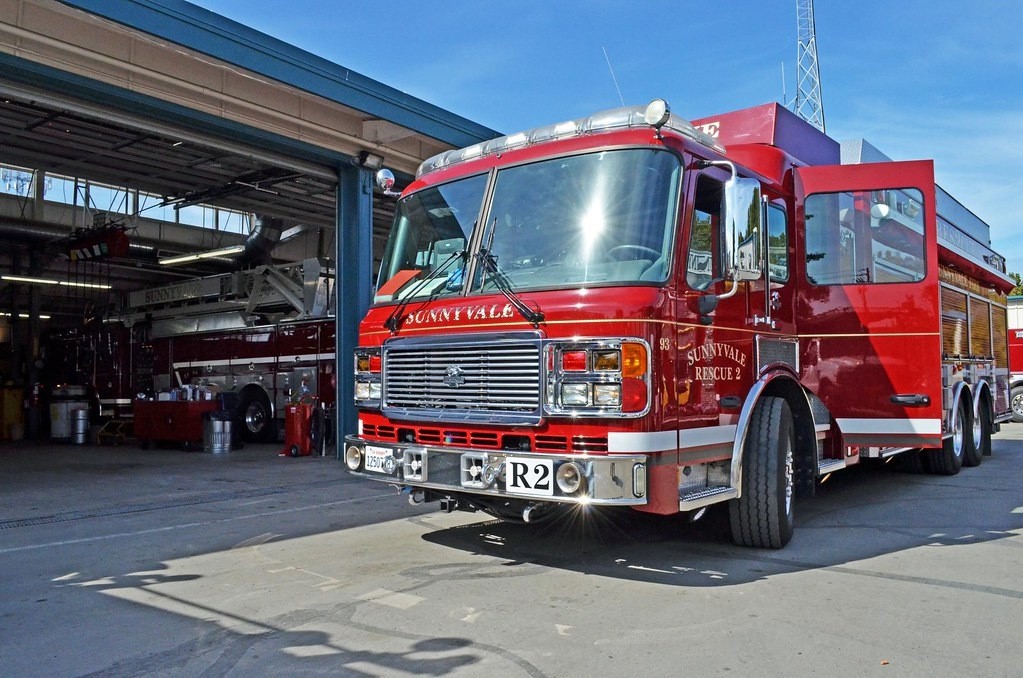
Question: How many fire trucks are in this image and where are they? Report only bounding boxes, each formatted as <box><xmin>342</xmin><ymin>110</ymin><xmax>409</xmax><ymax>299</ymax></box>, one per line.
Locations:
<box><xmin>83</xmin><ymin>256</ymin><xmax>339</xmax><ymax>445</ymax></box>
<box><xmin>341</xmin><ymin>98</ymin><xmax>1017</xmax><ymax>549</ymax></box>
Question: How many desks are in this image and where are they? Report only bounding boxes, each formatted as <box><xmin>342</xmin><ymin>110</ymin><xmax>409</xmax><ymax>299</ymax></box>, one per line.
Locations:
<box><xmin>133</xmin><ymin>399</ymin><xmax>225</xmax><ymax>452</ymax></box>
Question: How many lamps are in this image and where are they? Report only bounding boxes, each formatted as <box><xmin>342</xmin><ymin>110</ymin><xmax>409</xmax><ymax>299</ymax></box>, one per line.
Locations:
<box><xmin>358</xmin><ymin>150</ymin><xmax>385</xmax><ymax>172</ymax></box>
<box><xmin>158</xmin><ymin>243</ymin><xmax>246</xmax><ymax>265</ymax></box>
<box><xmin>59</xmin><ymin>279</ymin><xmax>113</xmax><ymax>290</ymax></box>
<box><xmin>0</xmin><ymin>274</ymin><xmax>59</xmax><ymax>285</ymax></box>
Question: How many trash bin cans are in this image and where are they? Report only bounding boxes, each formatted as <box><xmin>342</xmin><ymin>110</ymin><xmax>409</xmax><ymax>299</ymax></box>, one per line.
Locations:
<box><xmin>71</xmin><ymin>409</ymin><xmax>89</xmax><ymax>445</ymax></box>
<box><xmin>202</xmin><ymin>410</ymin><xmax>232</xmax><ymax>455</ymax></box>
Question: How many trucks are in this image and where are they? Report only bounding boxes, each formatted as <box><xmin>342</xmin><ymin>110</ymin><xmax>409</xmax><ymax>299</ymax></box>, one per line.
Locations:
<box><xmin>1009</xmin><ymin>328</ymin><xmax>1023</xmax><ymax>424</ymax></box>
